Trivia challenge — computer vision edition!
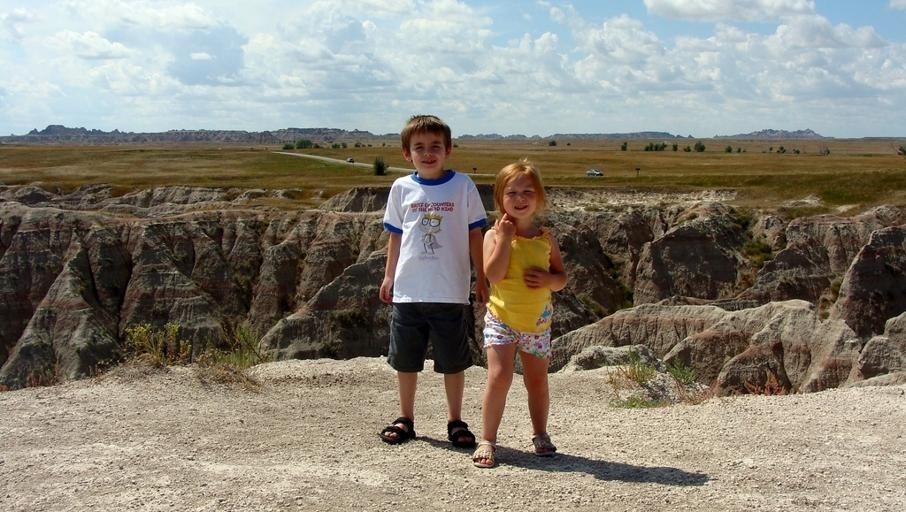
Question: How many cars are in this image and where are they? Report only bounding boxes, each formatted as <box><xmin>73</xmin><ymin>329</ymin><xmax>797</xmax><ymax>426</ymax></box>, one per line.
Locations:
<box><xmin>346</xmin><ymin>158</ymin><xmax>354</xmax><ymax>163</ymax></box>
<box><xmin>586</xmin><ymin>169</ymin><xmax>603</xmax><ymax>176</ymax></box>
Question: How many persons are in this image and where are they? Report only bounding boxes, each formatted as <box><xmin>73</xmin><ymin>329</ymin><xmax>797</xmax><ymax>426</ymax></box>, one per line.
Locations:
<box><xmin>379</xmin><ymin>114</ymin><xmax>489</xmax><ymax>448</ymax></box>
<box><xmin>473</xmin><ymin>160</ymin><xmax>568</xmax><ymax>469</ymax></box>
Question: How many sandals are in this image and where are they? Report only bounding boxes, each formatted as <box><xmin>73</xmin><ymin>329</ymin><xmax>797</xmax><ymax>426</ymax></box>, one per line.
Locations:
<box><xmin>379</xmin><ymin>418</ymin><xmax>416</xmax><ymax>442</ymax></box>
<box><xmin>531</xmin><ymin>432</ymin><xmax>556</xmax><ymax>456</ymax></box>
<box><xmin>448</xmin><ymin>418</ymin><xmax>476</xmax><ymax>448</ymax></box>
<box><xmin>472</xmin><ymin>441</ymin><xmax>497</xmax><ymax>467</ymax></box>
